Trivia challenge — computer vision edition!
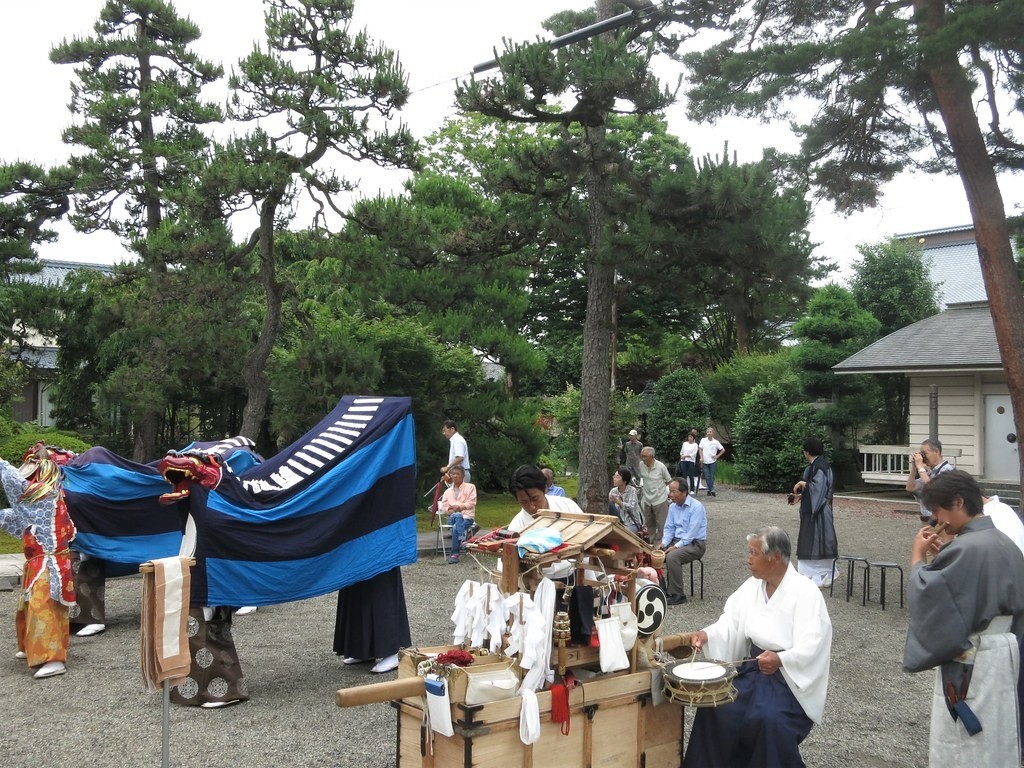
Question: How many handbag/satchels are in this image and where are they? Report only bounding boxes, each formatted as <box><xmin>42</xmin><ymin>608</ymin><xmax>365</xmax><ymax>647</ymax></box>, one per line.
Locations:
<box><xmin>675</xmin><ymin>462</ymin><xmax>682</xmax><ymax>476</ymax></box>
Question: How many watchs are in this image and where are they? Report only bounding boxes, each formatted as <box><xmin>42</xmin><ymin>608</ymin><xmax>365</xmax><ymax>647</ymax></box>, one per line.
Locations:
<box><xmin>918</xmin><ymin>468</ymin><xmax>925</xmax><ymax>474</ymax></box>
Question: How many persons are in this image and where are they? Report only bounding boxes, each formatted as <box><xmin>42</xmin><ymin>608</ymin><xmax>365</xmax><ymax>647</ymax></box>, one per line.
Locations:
<box><xmin>977</xmin><ymin>488</ymin><xmax>1024</xmax><ymax>559</ymax></box>
<box><xmin>639</xmin><ymin>446</ymin><xmax>673</xmax><ymax>550</ymax></box>
<box><xmin>905</xmin><ymin>438</ymin><xmax>954</xmax><ymax>566</ymax></box>
<box><xmin>439</xmin><ymin>466</ymin><xmax>477</xmax><ymax>564</ymax></box>
<box><xmin>0</xmin><ymin>453</ymin><xmax>75</xmax><ymax>679</ymax></box>
<box><xmin>507</xmin><ymin>464</ymin><xmax>589</xmax><ymax>613</ymax></box>
<box><xmin>900</xmin><ymin>470</ymin><xmax>1024</xmax><ymax>768</ymax></box>
<box><xmin>607</xmin><ymin>469</ymin><xmax>646</xmax><ymax>536</ymax></box>
<box><xmin>333</xmin><ymin>567</ymin><xmax>413</xmax><ymax>675</ymax></box>
<box><xmin>66</xmin><ymin>550</ymin><xmax>106</xmax><ymax>637</ymax></box>
<box><xmin>171</xmin><ymin>499</ymin><xmax>251</xmax><ymax>708</ymax></box>
<box><xmin>788</xmin><ymin>438</ymin><xmax>840</xmax><ymax>588</ymax></box>
<box><xmin>680</xmin><ymin>526</ymin><xmax>832</xmax><ymax>768</ymax></box>
<box><xmin>541</xmin><ymin>468</ymin><xmax>565</xmax><ymax>497</ymax></box>
<box><xmin>679</xmin><ymin>427</ymin><xmax>725</xmax><ymax>497</ymax></box>
<box><xmin>623</xmin><ymin>430</ymin><xmax>644</xmax><ymax>504</ymax></box>
<box><xmin>658</xmin><ymin>477</ymin><xmax>707</xmax><ymax>605</ymax></box>
<box><xmin>439</xmin><ymin>419</ymin><xmax>481</xmax><ymax>541</ymax></box>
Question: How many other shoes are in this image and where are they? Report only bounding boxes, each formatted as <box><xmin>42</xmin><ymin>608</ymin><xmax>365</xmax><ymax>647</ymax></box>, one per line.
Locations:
<box><xmin>448</xmin><ymin>555</ymin><xmax>459</xmax><ymax>564</ymax></box>
<box><xmin>461</xmin><ymin>540</ymin><xmax>468</xmax><ymax>550</ymax></box>
<box><xmin>201</xmin><ymin>699</ymin><xmax>239</xmax><ymax>709</ymax></box>
<box><xmin>690</xmin><ymin>491</ymin><xmax>694</xmax><ymax>494</ymax></box>
<box><xmin>16</xmin><ymin>651</ymin><xmax>28</xmax><ymax>659</ymax></box>
<box><xmin>707</xmin><ymin>492</ymin><xmax>711</xmax><ymax>496</ymax></box>
<box><xmin>712</xmin><ymin>492</ymin><xmax>716</xmax><ymax>496</ymax></box>
<box><xmin>76</xmin><ymin>624</ymin><xmax>105</xmax><ymax>636</ymax></box>
<box><xmin>821</xmin><ymin>572</ymin><xmax>840</xmax><ymax>587</ymax></box>
<box><xmin>34</xmin><ymin>661</ymin><xmax>66</xmax><ymax>678</ymax></box>
<box><xmin>343</xmin><ymin>656</ymin><xmax>375</xmax><ymax>664</ymax></box>
<box><xmin>235</xmin><ymin>606</ymin><xmax>256</xmax><ymax>616</ymax></box>
<box><xmin>371</xmin><ymin>653</ymin><xmax>398</xmax><ymax>673</ymax></box>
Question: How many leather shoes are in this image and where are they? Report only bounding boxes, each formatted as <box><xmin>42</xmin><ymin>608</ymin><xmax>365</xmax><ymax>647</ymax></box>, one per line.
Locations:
<box><xmin>666</xmin><ymin>594</ymin><xmax>686</xmax><ymax>605</ymax></box>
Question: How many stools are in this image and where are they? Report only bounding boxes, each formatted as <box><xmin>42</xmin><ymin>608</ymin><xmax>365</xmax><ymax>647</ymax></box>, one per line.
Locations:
<box><xmin>666</xmin><ymin>558</ymin><xmax>703</xmax><ymax>600</ymax></box>
<box><xmin>863</xmin><ymin>561</ymin><xmax>903</xmax><ymax>610</ymax></box>
<box><xmin>831</xmin><ymin>555</ymin><xmax>870</xmax><ymax>602</ymax></box>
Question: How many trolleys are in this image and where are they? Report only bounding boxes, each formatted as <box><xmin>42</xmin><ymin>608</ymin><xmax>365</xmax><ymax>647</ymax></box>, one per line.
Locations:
<box><xmin>334</xmin><ymin>509</ymin><xmax>684</xmax><ymax>768</ymax></box>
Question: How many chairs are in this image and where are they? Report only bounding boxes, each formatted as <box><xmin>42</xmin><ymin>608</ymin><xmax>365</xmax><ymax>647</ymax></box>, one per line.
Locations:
<box><xmin>435</xmin><ymin>500</ymin><xmax>475</xmax><ymax>561</ymax></box>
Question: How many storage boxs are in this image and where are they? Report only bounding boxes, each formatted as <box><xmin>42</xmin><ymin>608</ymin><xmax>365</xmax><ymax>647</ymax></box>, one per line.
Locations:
<box><xmin>397</xmin><ymin>643</ymin><xmax>521</xmax><ymax>708</ymax></box>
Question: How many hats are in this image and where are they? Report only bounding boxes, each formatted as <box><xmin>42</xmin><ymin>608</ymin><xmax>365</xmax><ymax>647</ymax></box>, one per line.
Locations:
<box><xmin>628</xmin><ymin>429</ymin><xmax>637</xmax><ymax>436</ymax></box>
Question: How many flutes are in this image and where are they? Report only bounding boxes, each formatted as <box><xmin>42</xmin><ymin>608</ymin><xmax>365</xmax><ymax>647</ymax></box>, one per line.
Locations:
<box><xmin>935</xmin><ymin>521</ymin><xmax>951</xmax><ymax>534</ymax></box>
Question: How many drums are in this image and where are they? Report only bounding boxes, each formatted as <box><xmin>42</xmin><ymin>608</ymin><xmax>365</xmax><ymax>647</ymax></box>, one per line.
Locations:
<box><xmin>658</xmin><ymin>657</ymin><xmax>739</xmax><ymax>707</ymax></box>
